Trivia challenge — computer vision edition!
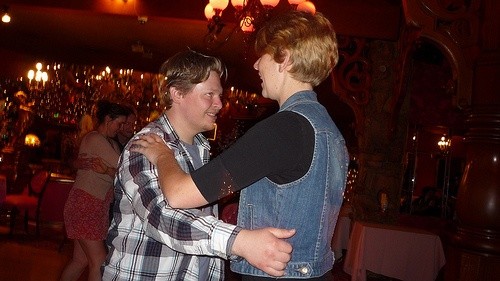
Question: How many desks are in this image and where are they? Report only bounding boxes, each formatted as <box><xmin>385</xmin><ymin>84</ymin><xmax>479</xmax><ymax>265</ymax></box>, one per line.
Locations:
<box><xmin>344</xmin><ymin>221</ymin><xmax>445</xmax><ymax>281</ymax></box>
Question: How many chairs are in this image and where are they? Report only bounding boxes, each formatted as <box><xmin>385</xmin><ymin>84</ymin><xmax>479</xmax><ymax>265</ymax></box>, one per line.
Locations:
<box><xmin>0</xmin><ymin>168</ymin><xmax>51</xmax><ymax>240</ymax></box>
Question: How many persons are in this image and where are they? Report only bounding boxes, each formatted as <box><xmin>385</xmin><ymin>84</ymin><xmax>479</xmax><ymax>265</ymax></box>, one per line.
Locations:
<box><xmin>58</xmin><ymin>100</ymin><xmax>138</xmax><ymax>281</ymax></box>
<box><xmin>100</xmin><ymin>48</ymin><xmax>295</xmax><ymax>281</ymax></box>
<box><xmin>129</xmin><ymin>8</ymin><xmax>356</xmax><ymax>281</ymax></box>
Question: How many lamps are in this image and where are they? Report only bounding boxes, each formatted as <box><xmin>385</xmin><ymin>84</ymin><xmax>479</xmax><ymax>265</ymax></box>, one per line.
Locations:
<box><xmin>204</xmin><ymin>0</ymin><xmax>316</xmax><ymax>62</ymax></box>
